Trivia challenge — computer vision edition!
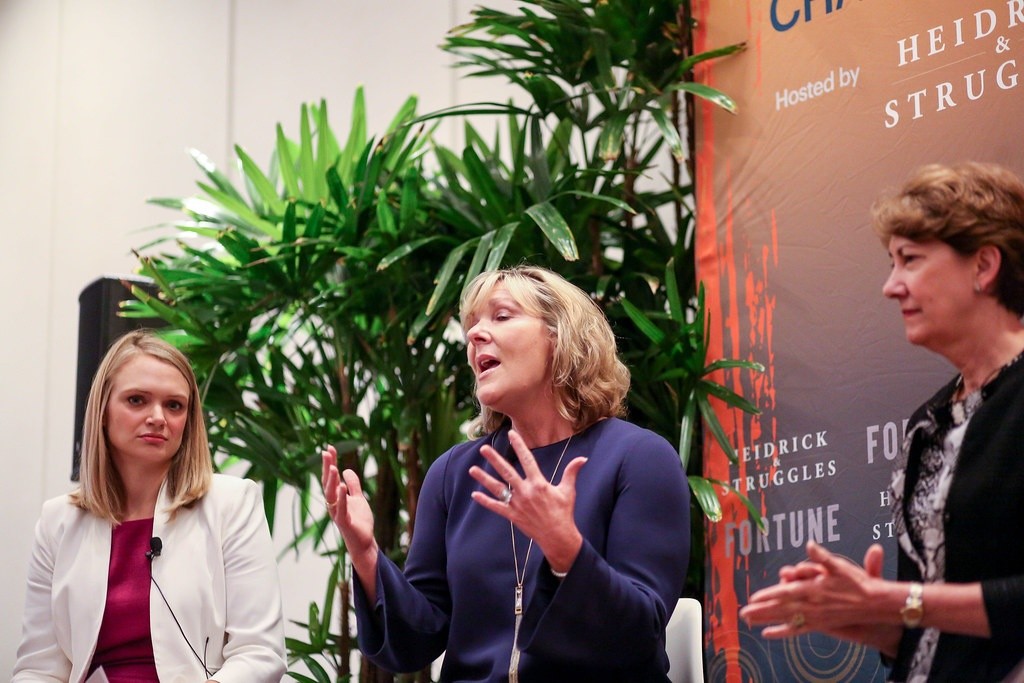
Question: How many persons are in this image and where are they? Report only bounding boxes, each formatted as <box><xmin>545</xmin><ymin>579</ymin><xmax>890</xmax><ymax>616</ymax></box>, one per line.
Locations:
<box><xmin>739</xmin><ymin>162</ymin><xmax>1024</xmax><ymax>683</ymax></box>
<box><xmin>320</xmin><ymin>264</ymin><xmax>692</xmax><ymax>683</ymax></box>
<box><xmin>9</xmin><ymin>329</ymin><xmax>288</xmax><ymax>683</ymax></box>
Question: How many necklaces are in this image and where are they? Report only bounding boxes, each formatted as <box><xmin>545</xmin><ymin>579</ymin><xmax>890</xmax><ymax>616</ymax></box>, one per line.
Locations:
<box><xmin>505</xmin><ymin>429</ymin><xmax>575</xmax><ymax>615</ymax></box>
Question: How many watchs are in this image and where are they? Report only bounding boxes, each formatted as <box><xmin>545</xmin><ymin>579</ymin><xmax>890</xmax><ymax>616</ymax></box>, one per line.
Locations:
<box><xmin>899</xmin><ymin>581</ymin><xmax>923</xmax><ymax>628</ymax></box>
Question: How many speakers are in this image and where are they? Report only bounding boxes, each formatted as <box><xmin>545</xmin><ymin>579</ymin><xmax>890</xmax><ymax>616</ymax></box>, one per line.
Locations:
<box><xmin>71</xmin><ymin>278</ymin><xmax>167</xmax><ymax>480</ymax></box>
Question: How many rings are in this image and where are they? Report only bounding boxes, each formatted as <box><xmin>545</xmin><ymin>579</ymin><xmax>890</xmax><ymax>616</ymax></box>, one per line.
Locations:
<box><xmin>793</xmin><ymin>613</ymin><xmax>805</xmax><ymax>628</ymax></box>
<box><xmin>500</xmin><ymin>488</ymin><xmax>512</xmax><ymax>504</ymax></box>
<box><xmin>324</xmin><ymin>500</ymin><xmax>338</xmax><ymax>511</ymax></box>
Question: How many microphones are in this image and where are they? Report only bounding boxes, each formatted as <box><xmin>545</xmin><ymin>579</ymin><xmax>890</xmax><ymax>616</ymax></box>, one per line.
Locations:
<box><xmin>145</xmin><ymin>537</ymin><xmax>162</xmax><ymax>560</ymax></box>
<box><xmin>506</xmin><ymin>445</ymin><xmax>519</xmax><ymax>467</ymax></box>
